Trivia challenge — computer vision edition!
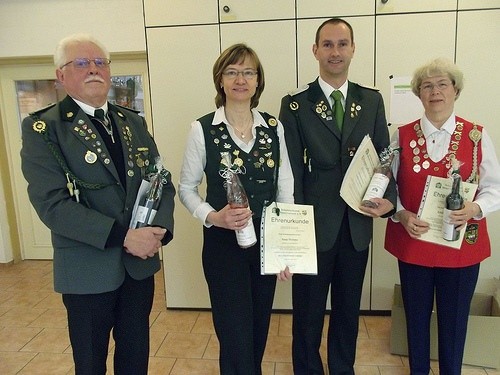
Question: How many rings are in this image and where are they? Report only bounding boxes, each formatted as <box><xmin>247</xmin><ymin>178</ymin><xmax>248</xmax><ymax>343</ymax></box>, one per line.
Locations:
<box><xmin>413</xmin><ymin>227</ymin><xmax>416</xmax><ymax>231</ymax></box>
<box><xmin>236</xmin><ymin>222</ymin><xmax>237</xmax><ymax>226</ymax></box>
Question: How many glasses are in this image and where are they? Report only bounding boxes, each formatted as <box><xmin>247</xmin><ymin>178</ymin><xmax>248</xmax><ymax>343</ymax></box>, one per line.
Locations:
<box><xmin>59</xmin><ymin>56</ymin><xmax>111</xmax><ymax>70</ymax></box>
<box><xmin>419</xmin><ymin>80</ymin><xmax>453</xmax><ymax>92</ymax></box>
<box><xmin>223</xmin><ymin>70</ymin><xmax>258</xmax><ymax>78</ymax></box>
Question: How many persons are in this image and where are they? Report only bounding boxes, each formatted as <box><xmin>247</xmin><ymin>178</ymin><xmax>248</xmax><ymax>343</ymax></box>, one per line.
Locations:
<box><xmin>177</xmin><ymin>43</ymin><xmax>295</xmax><ymax>375</ymax></box>
<box><xmin>384</xmin><ymin>59</ymin><xmax>500</xmax><ymax>375</ymax></box>
<box><xmin>279</xmin><ymin>17</ymin><xmax>398</xmax><ymax>375</ymax></box>
<box><xmin>20</xmin><ymin>35</ymin><xmax>176</xmax><ymax>375</ymax></box>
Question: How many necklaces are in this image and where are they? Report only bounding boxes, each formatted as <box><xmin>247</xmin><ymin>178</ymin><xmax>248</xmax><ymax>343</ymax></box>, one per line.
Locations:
<box><xmin>233</xmin><ymin>117</ymin><xmax>252</xmax><ymax>138</ymax></box>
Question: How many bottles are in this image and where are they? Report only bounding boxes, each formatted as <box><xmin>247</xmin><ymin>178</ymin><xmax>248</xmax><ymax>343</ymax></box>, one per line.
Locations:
<box><xmin>134</xmin><ymin>172</ymin><xmax>164</xmax><ymax>229</ymax></box>
<box><xmin>442</xmin><ymin>175</ymin><xmax>462</xmax><ymax>241</ymax></box>
<box><xmin>361</xmin><ymin>150</ymin><xmax>396</xmax><ymax>208</ymax></box>
<box><xmin>225</xmin><ymin>166</ymin><xmax>257</xmax><ymax>248</ymax></box>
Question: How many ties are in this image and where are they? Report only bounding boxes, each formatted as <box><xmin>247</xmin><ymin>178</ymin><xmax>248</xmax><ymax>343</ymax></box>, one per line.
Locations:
<box><xmin>94</xmin><ymin>109</ymin><xmax>111</xmax><ymax>132</ymax></box>
<box><xmin>331</xmin><ymin>90</ymin><xmax>344</xmax><ymax>134</ymax></box>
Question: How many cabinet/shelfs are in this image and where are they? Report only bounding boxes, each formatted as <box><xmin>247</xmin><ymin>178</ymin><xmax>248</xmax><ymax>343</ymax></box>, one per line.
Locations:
<box><xmin>143</xmin><ymin>0</ymin><xmax>499</xmax><ymax>317</ymax></box>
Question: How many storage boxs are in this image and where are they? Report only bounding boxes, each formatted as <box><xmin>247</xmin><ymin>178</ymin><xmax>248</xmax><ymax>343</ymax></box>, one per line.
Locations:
<box><xmin>390</xmin><ymin>282</ymin><xmax>500</xmax><ymax>371</ymax></box>
